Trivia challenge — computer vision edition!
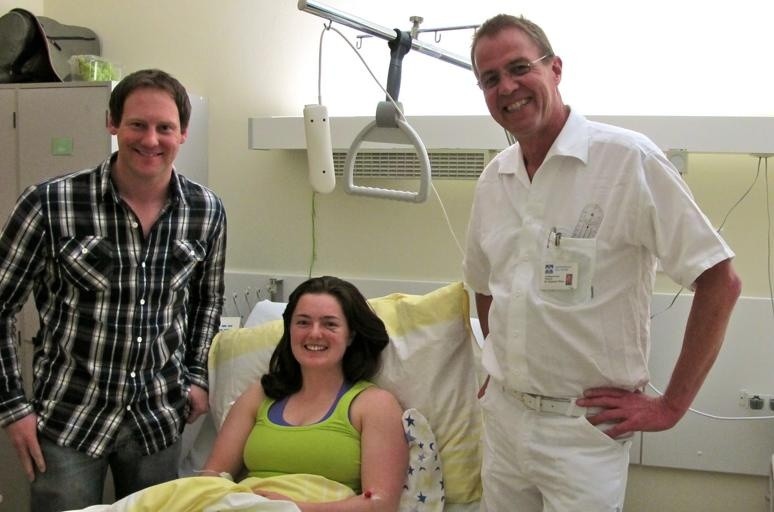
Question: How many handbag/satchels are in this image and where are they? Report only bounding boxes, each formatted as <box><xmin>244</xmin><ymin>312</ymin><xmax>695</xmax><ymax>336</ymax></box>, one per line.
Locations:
<box><xmin>0</xmin><ymin>8</ymin><xmax>101</xmax><ymax>81</ymax></box>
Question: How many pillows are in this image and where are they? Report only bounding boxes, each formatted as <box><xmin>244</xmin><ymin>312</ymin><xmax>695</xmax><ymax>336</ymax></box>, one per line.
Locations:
<box><xmin>207</xmin><ymin>281</ymin><xmax>484</xmax><ymax>504</ymax></box>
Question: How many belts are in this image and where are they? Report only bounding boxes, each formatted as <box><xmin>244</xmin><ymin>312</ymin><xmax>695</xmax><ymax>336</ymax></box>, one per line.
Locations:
<box><xmin>490</xmin><ymin>376</ymin><xmax>604</xmax><ymax>417</ymax></box>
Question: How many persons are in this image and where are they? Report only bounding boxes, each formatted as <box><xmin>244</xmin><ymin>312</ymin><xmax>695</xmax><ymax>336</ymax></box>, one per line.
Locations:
<box><xmin>461</xmin><ymin>13</ymin><xmax>742</xmax><ymax>512</ymax></box>
<box><xmin>200</xmin><ymin>276</ymin><xmax>410</xmax><ymax>512</ymax></box>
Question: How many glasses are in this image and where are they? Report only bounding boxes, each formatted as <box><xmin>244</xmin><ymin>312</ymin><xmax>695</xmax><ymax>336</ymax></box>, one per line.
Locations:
<box><xmin>476</xmin><ymin>54</ymin><xmax>548</xmax><ymax>91</ymax></box>
